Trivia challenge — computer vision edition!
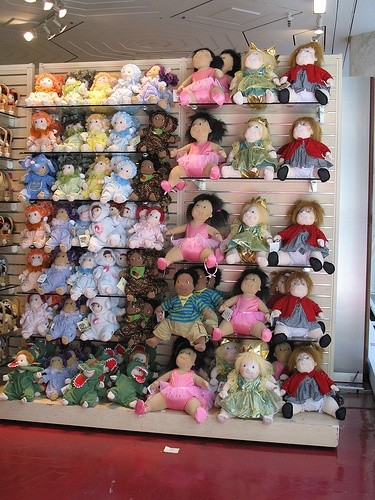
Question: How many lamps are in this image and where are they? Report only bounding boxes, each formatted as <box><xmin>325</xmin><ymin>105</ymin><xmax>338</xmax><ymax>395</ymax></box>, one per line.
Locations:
<box><xmin>315</xmin><ymin>17</ymin><xmax>324</xmax><ymax>34</ymax></box>
<box><xmin>23</xmin><ymin>0</ymin><xmax>69</xmax><ymax>43</ymax></box>
<box><xmin>311</xmin><ymin>34</ymin><xmax>323</xmax><ymax>43</ymax></box>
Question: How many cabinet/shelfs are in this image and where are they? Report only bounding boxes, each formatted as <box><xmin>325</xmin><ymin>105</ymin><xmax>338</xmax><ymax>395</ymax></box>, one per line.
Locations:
<box><xmin>171</xmin><ymin>99</ymin><xmax>323</xmax><ymax>343</ymax></box>
<box><xmin>0</xmin><ymin>110</ymin><xmax>19</xmax><ymax>309</ymax></box>
<box><xmin>23</xmin><ymin>100</ymin><xmax>164</xmax><ymax>344</ymax></box>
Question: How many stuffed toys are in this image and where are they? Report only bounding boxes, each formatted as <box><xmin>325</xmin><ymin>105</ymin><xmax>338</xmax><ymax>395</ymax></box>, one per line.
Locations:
<box><xmin>222</xmin><ymin>116</ymin><xmax>278</xmax><ymax>181</ymax></box>
<box><xmin>276</xmin><ymin>117</ymin><xmax>333</xmax><ymax>183</ymax></box>
<box><xmin>145</xmin><ymin>267</ymin><xmax>225</xmax><ymax>352</ymax></box>
<box><xmin>268</xmin><ymin>200</ymin><xmax>336</xmax><ymax>275</ymax></box>
<box><xmin>229</xmin><ymin>42</ymin><xmax>280</xmax><ymax>105</ymax></box>
<box><xmin>0</xmin><ymin>64</ymin><xmax>180</xmax><ymax>409</ymax></box>
<box><xmin>160</xmin><ymin>113</ymin><xmax>227</xmax><ymax>192</ymax></box>
<box><xmin>213</xmin><ymin>269</ymin><xmax>272</xmax><ymax>342</ymax></box>
<box><xmin>272</xmin><ymin>342</ymin><xmax>347</xmax><ymax>419</ymax></box>
<box><xmin>136</xmin><ymin>337</ymin><xmax>216</xmax><ymax>422</ymax></box>
<box><xmin>207</xmin><ymin>338</ymin><xmax>281</xmax><ymax>424</ymax></box>
<box><xmin>157</xmin><ymin>194</ymin><xmax>230</xmax><ymax>270</ymax></box>
<box><xmin>266</xmin><ymin>270</ymin><xmax>332</xmax><ymax>348</ymax></box>
<box><xmin>278</xmin><ymin>42</ymin><xmax>333</xmax><ymax>105</ymax></box>
<box><xmin>177</xmin><ymin>48</ymin><xmax>241</xmax><ymax>106</ymax></box>
<box><xmin>222</xmin><ymin>196</ymin><xmax>273</xmax><ymax>267</ymax></box>
